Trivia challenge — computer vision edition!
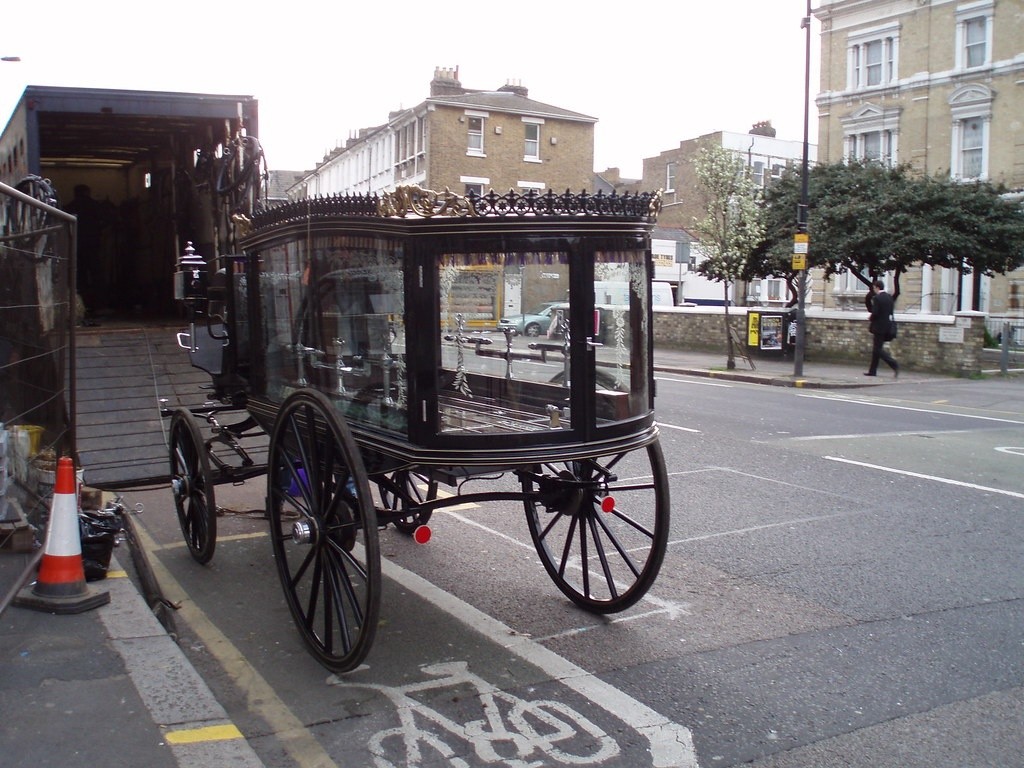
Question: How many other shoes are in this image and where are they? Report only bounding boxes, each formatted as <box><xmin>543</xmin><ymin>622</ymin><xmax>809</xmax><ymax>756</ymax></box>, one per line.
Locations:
<box><xmin>864</xmin><ymin>372</ymin><xmax>875</xmax><ymax>376</ymax></box>
<box><xmin>894</xmin><ymin>365</ymin><xmax>899</xmax><ymax>378</ymax></box>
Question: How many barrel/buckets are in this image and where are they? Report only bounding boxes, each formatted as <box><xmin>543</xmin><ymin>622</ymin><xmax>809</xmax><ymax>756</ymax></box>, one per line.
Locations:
<box><xmin>6</xmin><ymin>425</ymin><xmax>45</xmax><ymax>457</ymax></box>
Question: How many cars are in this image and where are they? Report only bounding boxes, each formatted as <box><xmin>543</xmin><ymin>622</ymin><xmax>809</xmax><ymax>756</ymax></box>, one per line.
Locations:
<box><xmin>495</xmin><ymin>298</ymin><xmax>571</xmax><ymax>338</ymax></box>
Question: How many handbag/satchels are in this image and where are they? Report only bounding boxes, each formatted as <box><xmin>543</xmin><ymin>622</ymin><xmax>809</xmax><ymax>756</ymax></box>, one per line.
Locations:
<box><xmin>884</xmin><ymin>320</ymin><xmax>897</xmax><ymax>341</ymax></box>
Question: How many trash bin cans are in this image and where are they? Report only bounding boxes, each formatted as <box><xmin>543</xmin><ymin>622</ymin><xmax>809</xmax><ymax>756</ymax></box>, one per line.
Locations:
<box><xmin>79</xmin><ymin>506</ymin><xmax>125</xmax><ymax>582</ymax></box>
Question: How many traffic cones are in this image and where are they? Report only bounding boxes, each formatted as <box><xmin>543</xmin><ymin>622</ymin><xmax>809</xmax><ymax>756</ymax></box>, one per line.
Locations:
<box><xmin>10</xmin><ymin>454</ymin><xmax>111</xmax><ymax>616</ymax></box>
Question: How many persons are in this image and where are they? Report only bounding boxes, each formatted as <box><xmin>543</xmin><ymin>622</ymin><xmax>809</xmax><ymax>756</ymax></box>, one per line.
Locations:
<box><xmin>64</xmin><ymin>185</ymin><xmax>110</xmax><ymax>322</ymax></box>
<box><xmin>864</xmin><ymin>281</ymin><xmax>900</xmax><ymax>377</ymax></box>
